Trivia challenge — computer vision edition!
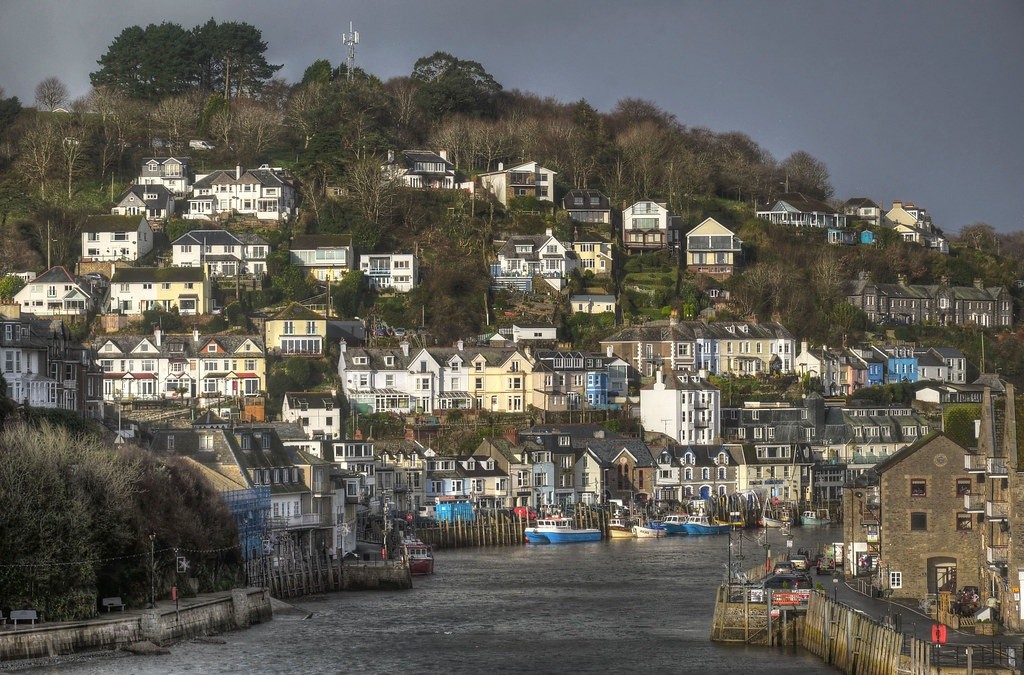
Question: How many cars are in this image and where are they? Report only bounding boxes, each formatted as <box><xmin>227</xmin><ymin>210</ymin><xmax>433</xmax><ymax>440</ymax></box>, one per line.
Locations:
<box><xmin>773</xmin><ymin>562</ymin><xmax>797</xmax><ymax>575</ymax></box>
<box><xmin>816</xmin><ymin>557</ymin><xmax>836</xmax><ymax>575</ymax></box>
<box><xmin>768</xmin><ymin>574</ymin><xmax>814</xmax><ymax>613</ymax></box>
<box><xmin>789</xmin><ymin>554</ymin><xmax>810</xmax><ymax>572</ymax></box>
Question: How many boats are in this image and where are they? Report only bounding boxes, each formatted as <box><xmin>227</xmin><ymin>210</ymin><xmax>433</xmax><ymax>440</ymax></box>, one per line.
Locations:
<box><xmin>762</xmin><ymin>515</ymin><xmax>790</xmax><ymax>527</ymax></box>
<box><xmin>524</xmin><ymin>514</ymin><xmax>602</xmax><ymax>543</ymax></box>
<box><xmin>631</xmin><ymin>517</ymin><xmax>668</xmax><ymax>539</ymax></box>
<box><xmin>800</xmin><ymin>508</ymin><xmax>832</xmax><ymax>526</ymax></box>
<box><xmin>655</xmin><ymin>512</ymin><xmax>689</xmax><ymax>534</ymax></box>
<box><xmin>403</xmin><ymin>535</ymin><xmax>433</xmax><ymax>575</ymax></box>
<box><xmin>606</xmin><ymin>509</ymin><xmax>632</xmax><ymax>539</ymax></box>
<box><xmin>681</xmin><ymin>513</ymin><xmax>731</xmax><ymax>535</ymax></box>
